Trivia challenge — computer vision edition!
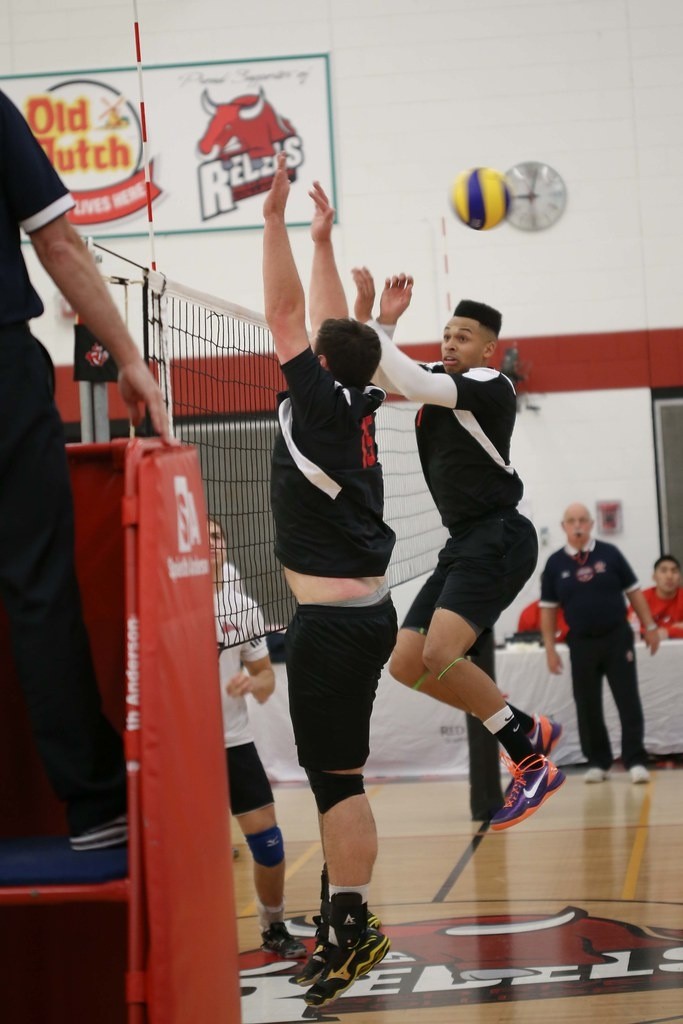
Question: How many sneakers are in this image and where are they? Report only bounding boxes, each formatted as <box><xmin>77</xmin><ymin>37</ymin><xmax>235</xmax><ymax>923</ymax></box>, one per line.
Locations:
<box><xmin>525</xmin><ymin>713</ymin><xmax>563</xmax><ymax>758</ymax></box>
<box><xmin>288</xmin><ymin>909</ymin><xmax>382</xmax><ymax>987</ymax></box>
<box><xmin>584</xmin><ymin>767</ymin><xmax>608</xmax><ymax>783</ymax></box>
<box><xmin>304</xmin><ymin>925</ymin><xmax>390</xmax><ymax>1009</ymax></box>
<box><xmin>261</xmin><ymin>922</ymin><xmax>307</xmax><ymax>958</ymax></box>
<box><xmin>629</xmin><ymin>763</ymin><xmax>650</xmax><ymax>782</ymax></box>
<box><xmin>489</xmin><ymin>752</ymin><xmax>567</xmax><ymax>831</ymax></box>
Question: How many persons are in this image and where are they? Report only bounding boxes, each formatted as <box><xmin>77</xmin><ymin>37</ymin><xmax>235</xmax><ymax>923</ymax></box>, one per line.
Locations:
<box><xmin>516</xmin><ymin>503</ymin><xmax>683</xmax><ymax>783</ymax></box>
<box><xmin>207</xmin><ymin>519</ymin><xmax>306</xmax><ymax>959</ymax></box>
<box><xmin>350</xmin><ymin>265</ymin><xmax>567</xmax><ymax>831</ymax></box>
<box><xmin>1</xmin><ymin>88</ymin><xmax>182</xmax><ymax>854</ymax></box>
<box><xmin>261</xmin><ymin>150</ymin><xmax>399</xmax><ymax>1013</ymax></box>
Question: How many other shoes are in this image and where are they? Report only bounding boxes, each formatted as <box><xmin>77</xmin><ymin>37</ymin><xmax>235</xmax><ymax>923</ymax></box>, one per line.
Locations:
<box><xmin>69</xmin><ymin>816</ymin><xmax>128</xmax><ymax>852</ymax></box>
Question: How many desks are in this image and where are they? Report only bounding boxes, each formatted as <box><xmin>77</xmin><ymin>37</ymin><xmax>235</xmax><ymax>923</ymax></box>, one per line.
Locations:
<box><xmin>245</xmin><ymin>622</ymin><xmax>683</xmax><ymax>785</ymax></box>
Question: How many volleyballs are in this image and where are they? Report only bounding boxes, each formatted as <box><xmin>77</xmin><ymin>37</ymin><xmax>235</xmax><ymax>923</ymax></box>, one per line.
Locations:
<box><xmin>452</xmin><ymin>165</ymin><xmax>513</xmax><ymax>231</ymax></box>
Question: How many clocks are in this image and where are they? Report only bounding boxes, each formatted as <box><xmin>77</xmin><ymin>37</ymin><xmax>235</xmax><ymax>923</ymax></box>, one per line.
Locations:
<box><xmin>503</xmin><ymin>161</ymin><xmax>567</xmax><ymax>233</ymax></box>
